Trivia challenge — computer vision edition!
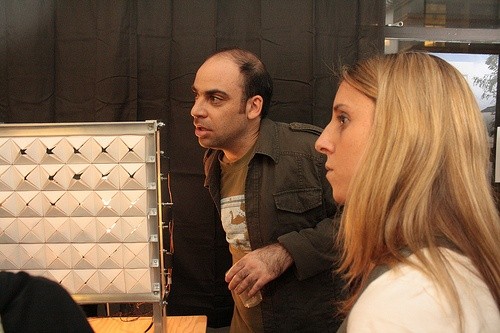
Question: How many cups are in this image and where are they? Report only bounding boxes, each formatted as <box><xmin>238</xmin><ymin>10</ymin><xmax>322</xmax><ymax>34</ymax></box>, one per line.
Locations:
<box><xmin>234</xmin><ymin>279</ymin><xmax>263</xmax><ymax>308</ymax></box>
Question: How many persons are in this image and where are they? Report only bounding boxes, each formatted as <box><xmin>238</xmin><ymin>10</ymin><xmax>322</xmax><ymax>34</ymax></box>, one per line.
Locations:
<box><xmin>0</xmin><ymin>272</ymin><xmax>95</xmax><ymax>333</ymax></box>
<box><xmin>315</xmin><ymin>51</ymin><xmax>500</xmax><ymax>333</ymax></box>
<box><xmin>191</xmin><ymin>47</ymin><xmax>355</xmax><ymax>333</ymax></box>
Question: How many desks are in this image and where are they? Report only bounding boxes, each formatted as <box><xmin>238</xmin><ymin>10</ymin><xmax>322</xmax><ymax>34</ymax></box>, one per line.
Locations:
<box><xmin>87</xmin><ymin>315</ymin><xmax>207</xmax><ymax>333</ymax></box>
